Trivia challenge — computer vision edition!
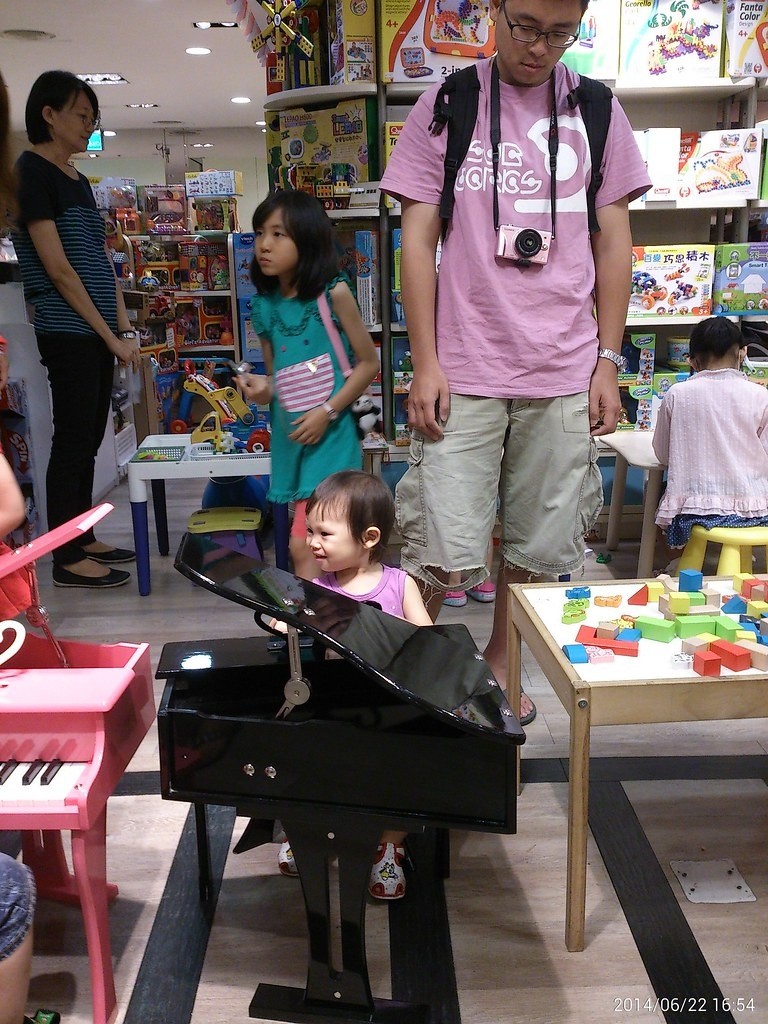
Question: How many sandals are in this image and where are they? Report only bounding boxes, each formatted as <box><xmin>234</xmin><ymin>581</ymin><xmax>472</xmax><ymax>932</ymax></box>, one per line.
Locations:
<box><xmin>277</xmin><ymin>837</ymin><xmax>300</xmax><ymax>877</ymax></box>
<box><xmin>368</xmin><ymin>843</ymin><xmax>406</xmax><ymax>899</ymax></box>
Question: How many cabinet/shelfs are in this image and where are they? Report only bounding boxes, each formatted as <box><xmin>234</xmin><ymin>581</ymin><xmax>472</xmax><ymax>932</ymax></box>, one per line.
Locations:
<box><xmin>259</xmin><ymin>82</ymin><xmax>385</xmax><ymax>463</ymax></box>
<box><xmin>133</xmin><ymin>236</ymin><xmax>238</xmax><ymax>434</ymax></box>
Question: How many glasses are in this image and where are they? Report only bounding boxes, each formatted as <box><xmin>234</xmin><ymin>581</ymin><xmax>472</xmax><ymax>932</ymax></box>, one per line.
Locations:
<box><xmin>501</xmin><ymin>0</ymin><xmax>581</xmax><ymax>48</ymax></box>
<box><xmin>59</xmin><ymin>109</ymin><xmax>101</xmax><ymax>129</ymax></box>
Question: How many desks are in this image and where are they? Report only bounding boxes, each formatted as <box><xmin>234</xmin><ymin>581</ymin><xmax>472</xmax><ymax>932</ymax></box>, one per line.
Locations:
<box><xmin>505</xmin><ymin>573</ymin><xmax>768</xmax><ymax>954</ymax></box>
<box><xmin>380</xmin><ymin>1</ymin><xmax>766</xmax><ymax>458</ymax></box>
<box><xmin>125</xmin><ymin>433</ymin><xmax>390</xmax><ymax>597</ymax></box>
<box><xmin>600</xmin><ymin>431</ymin><xmax>767</xmax><ymax>575</ymax></box>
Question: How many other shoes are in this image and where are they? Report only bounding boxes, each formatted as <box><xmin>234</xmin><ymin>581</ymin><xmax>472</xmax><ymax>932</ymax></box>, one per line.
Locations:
<box><xmin>443</xmin><ymin>587</ymin><xmax>467</xmax><ymax>607</ymax></box>
<box><xmin>86</xmin><ymin>548</ymin><xmax>135</xmax><ymax>562</ymax></box>
<box><xmin>51</xmin><ymin>560</ymin><xmax>132</xmax><ymax>588</ymax></box>
<box><xmin>467</xmin><ymin>581</ymin><xmax>496</xmax><ymax>602</ymax></box>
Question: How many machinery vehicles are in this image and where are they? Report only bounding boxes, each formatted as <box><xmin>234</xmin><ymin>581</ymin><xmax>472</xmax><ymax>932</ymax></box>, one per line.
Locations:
<box><xmin>184</xmin><ymin>373</ymin><xmax>270</xmax><ymax>455</ymax></box>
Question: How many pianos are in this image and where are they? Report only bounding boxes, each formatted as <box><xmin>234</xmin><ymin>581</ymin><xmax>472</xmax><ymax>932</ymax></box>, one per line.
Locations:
<box><xmin>0</xmin><ymin>502</ymin><xmax>156</xmax><ymax>1024</ymax></box>
<box><xmin>154</xmin><ymin>529</ymin><xmax>526</xmax><ymax>1024</ymax></box>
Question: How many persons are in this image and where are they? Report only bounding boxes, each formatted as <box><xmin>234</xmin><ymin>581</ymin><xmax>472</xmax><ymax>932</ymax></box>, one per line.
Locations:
<box><xmin>279</xmin><ymin>469</ymin><xmax>438</xmax><ymax>901</ymax></box>
<box><xmin>8</xmin><ymin>70</ymin><xmax>143</xmax><ymax>588</ymax></box>
<box><xmin>232</xmin><ymin>191</ymin><xmax>380</xmax><ymax>582</ymax></box>
<box><xmin>652</xmin><ymin>314</ymin><xmax>768</xmax><ymax>575</ymax></box>
<box><xmin>0</xmin><ymin>73</ymin><xmax>33</xmax><ymax>622</ymax></box>
<box><xmin>0</xmin><ymin>829</ymin><xmax>63</xmax><ymax>1024</ymax></box>
<box><xmin>378</xmin><ymin>0</ymin><xmax>654</xmax><ymax>726</ymax></box>
<box><xmin>443</xmin><ymin>531</ymin><xmax>498</xmax><ymax>607</ymax></box>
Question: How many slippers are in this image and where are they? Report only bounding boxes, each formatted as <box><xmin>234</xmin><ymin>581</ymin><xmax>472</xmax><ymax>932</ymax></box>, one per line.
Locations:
<box><xmin>503</xmin><ymin>685</ymin><xmax>537</xmax><ymax>725</ymax></box>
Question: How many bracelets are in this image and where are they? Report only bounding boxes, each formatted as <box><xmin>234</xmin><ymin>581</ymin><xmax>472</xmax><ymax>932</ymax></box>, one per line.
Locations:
<box><xmin>599</xmin><ymin>348</ymin><xmax>628</xmax><ymax>372</ymax></box>
<box><xmin>322</xmin><ymin>401</ymin><xmax>337</xmax><ymax>420</ymax></box>
<box><xmin>118</xmin><ymin>330</ymin><xmax>136</xmax><ymax>339</ymax></box>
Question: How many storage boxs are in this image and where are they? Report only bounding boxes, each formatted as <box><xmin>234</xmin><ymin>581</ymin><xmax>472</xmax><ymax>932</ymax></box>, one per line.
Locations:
<box><xmin>100</xmin><ymin>1</ymin><xmax>768</xmax><ymax>457</ymax></box>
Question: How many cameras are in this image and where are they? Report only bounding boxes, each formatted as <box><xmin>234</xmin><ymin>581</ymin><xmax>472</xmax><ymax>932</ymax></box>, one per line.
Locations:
<box><xmin>495</xmin><ymin>224</ymin><xmax>552</xmax><ymax>266</ymax></box>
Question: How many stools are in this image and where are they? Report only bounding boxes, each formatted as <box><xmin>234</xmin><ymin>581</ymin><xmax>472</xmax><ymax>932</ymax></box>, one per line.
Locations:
<box><xmin>175</xmin><ymin>509</ymin><xmax>267</xmax><ymax>571</ymax></box>
<box><xmin>678</xmin><ymin>521</ymin><xmax>767</xmax><ymax>582</ymax></box>
